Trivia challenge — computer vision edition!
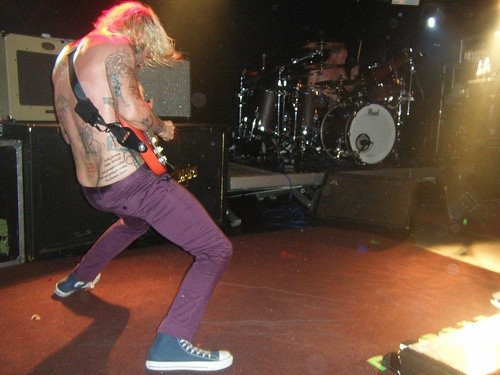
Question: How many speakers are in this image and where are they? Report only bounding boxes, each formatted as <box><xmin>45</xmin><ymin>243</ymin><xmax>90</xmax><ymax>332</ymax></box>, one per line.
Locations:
<box><xmin>0</xmin><ymin>34</ymin><xmax>230</xmax><ymax>268</ymax></box>
<box><xmin>310</xmin><ymin>171</ymin><xmax>419</xmax><ymax>231</ymax></box>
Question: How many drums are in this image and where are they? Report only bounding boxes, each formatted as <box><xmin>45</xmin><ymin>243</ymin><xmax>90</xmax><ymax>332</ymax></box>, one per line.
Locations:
<box><xmin>360</xmin><ymin>62</ymin><xmax>392</xmax><ymax>81</ymax></box>
<box><xmin>289</xmin><ymin>87</ymin><xmax>325</xmax><ymax>148</ymax></box>
<box><xmin>320</xmin><ymin>101</ymin><xmax>400</xmax><ymax>166</ymax></box>
<box><xmin>363</xmin><ymin>82</ymin><xmax>401</xmax><ymax>117</ymax></box>
<box><xmin>239</xmin><ymin>84</ymin><xmax>289</xmax><ymax>141</ymax></box>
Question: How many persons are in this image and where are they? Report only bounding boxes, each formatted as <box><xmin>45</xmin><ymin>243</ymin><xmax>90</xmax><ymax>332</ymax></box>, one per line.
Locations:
<box><xmin>52</xmin><ymin>0</ymin><xmax>234</xmax><ymax>371</ymax></box>
<box><xmin>388</xmin><ymin>46</ymin><xmax>423</xmax><ymax>117</ymax></box>
<box><xmin>307</xmin><ymin>45</ymin><xmax>359</xmax><ymax>102</ymax></box>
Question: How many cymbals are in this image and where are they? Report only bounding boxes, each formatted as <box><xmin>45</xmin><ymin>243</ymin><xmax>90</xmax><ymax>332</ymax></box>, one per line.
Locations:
<box><xmin>304</xmin><ymin>63</ymin><xmax>345</xmax><ymax>70</ymax></box>
<box><xmin>314</xmin><ymin>78</ymin><xmax>356</xmax><ymax>89</ymax></box>
<box><xmin>303</xmin><ymin>42</ymin><xmax>344</xmax><ymax>49</ymax></box>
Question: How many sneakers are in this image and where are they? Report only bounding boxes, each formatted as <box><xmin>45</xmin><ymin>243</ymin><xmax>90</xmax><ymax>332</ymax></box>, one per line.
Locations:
<box><xmin>54</xmin><ymin>273</ymin><xmax>101</xmax><ymax>297</ymax></box>
<box><xmin>146</xmin><ymin>333</ymin><xmax>233</xmax><ymax>371</ymax></box>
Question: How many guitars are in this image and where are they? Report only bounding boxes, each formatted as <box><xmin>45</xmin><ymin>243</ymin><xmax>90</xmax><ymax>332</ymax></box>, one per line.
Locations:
<box><xmin>115</xmin><ymin>95</ymin><xmax>167</xmax><ymax>176</ymax></box>
